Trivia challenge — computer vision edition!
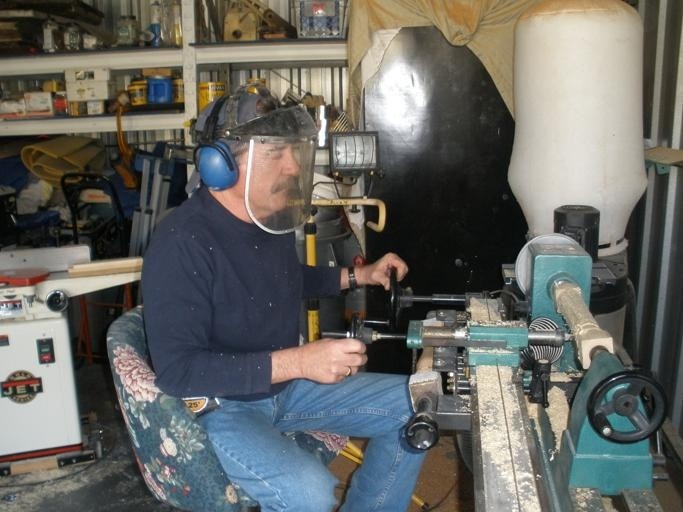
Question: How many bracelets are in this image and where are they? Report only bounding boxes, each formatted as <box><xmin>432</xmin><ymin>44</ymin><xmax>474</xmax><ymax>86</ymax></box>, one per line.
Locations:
<box><xmin>348</xmin><ymin>265</ymin><xmax>358</xmax><ymax>290</ymax></box>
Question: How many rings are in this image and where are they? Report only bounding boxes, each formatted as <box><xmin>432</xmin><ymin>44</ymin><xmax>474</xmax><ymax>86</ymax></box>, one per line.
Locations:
<box><xmin>344</xmin><ymin>365</ymin><xmax>352</xmax><ymax>378</ymax></box>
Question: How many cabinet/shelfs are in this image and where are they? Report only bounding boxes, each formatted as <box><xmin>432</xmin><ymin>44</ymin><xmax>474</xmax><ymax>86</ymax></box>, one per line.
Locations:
<box><xmin>0</xmin><ymin>1</ymin><xmax>367</xmax><ymax>201</ymax></box>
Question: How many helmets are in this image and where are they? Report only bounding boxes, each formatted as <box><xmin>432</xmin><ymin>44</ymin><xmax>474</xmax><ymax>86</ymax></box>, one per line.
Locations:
<box><xmin>190</xmin><ymin>92</ymin><xmax>319</xmax><ymax>235</ymax></box>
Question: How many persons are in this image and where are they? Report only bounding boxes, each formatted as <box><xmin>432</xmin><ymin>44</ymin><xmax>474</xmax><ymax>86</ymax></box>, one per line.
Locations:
<box><xmin>139</xmin><ymin>90</ymin><xmax>432</xmax><ymax>511</ymax></box>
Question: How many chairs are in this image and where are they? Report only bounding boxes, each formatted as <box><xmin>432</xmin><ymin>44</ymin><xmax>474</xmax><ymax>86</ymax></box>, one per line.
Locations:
<box><xmin>108</xmin><ymin>303</ymin><xmax>434</xmax><ymax>511</ymax></box>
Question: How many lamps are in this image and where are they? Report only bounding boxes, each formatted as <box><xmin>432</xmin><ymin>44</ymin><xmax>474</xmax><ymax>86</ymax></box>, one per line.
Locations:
<box><xmin>331</xmin><ymin>133</ymin><xmax>379</xmax><ymax>198</ymax></box>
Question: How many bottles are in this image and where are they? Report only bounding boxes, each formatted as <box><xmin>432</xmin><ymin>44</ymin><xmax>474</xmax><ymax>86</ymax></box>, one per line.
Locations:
<box><xmin>147</xmin><ymin>1</ymin><xmax>182</xmax><ymax>48</ymax></box>
<box><xmin>115</xmin><ymin>14</ymin><xmax>138</xmax><ymax>48</ymax></box>
<box><xmin>42</xmin><ymin>15</ymin><xmax>82</xmax><ymax>54</ymax></box>
